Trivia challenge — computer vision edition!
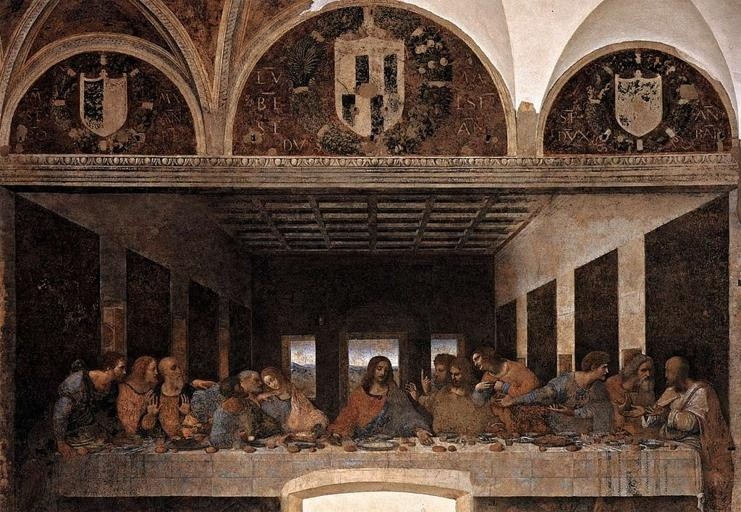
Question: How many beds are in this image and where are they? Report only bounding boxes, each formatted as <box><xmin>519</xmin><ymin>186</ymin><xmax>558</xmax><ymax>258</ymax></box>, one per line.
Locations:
<box><xmin>52</xmin><ymin>436</ymin><xmax>700</xmax><ymax>496</ymax></box>
